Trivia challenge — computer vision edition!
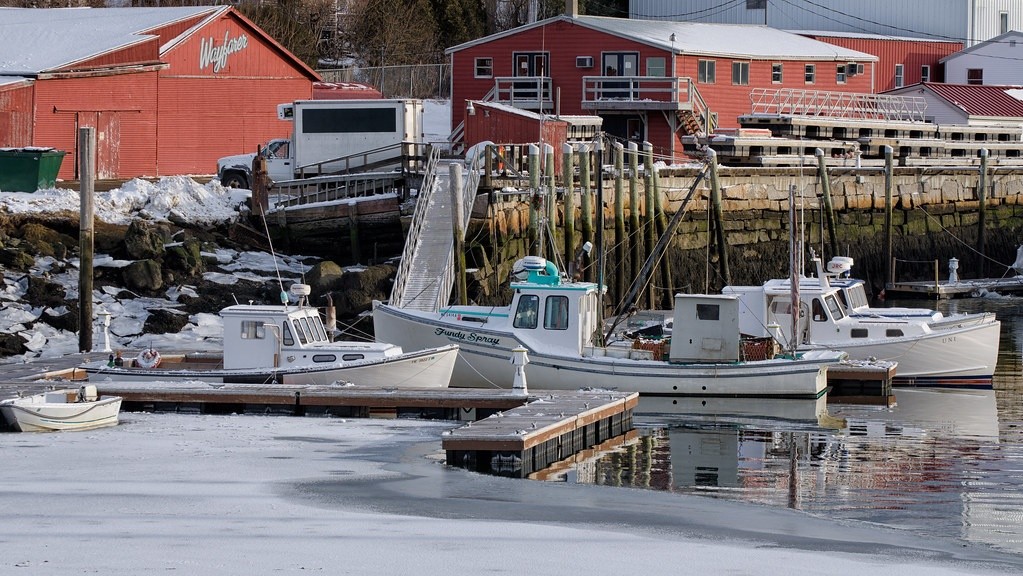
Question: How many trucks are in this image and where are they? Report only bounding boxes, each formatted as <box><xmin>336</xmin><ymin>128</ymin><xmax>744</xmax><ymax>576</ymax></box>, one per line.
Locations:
<box><xmin>217</xmin><ymin>99</ymin><xmax>453</xmax><ymax>191</ymax></box>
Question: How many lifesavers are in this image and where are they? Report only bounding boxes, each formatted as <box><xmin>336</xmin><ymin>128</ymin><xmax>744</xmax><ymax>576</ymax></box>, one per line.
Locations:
<box><xmin>137</xmin><ymin>349</ymin><xmax>161</xmax><ymax>368</ymax></box>
<box><xmin>512</xmin><ymin>255</ymin><xmax>558</xmax><ymax>280</ymax></box>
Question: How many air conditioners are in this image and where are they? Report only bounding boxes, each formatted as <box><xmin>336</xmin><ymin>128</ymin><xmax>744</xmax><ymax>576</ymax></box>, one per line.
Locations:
<box><xmin>576</xmin><ymin>56</ymin><xmax>594</xmax><ymax>68</ymax></box>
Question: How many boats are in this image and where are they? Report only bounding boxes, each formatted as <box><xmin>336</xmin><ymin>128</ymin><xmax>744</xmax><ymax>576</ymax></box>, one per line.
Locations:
<box><xmin>0</xmin><ymin>384</ymin><xmax>123</xmax><ymax>432</ymax></box>
<box><xmin>827</xmin><ymin>385</ymin><xmax>999</xmax><ymax>444</ymax></box>
<box><xmin>762</xmin><ymin>256</ymin><xmax>1000</xmax><ymax>389</ymax></box>
<box><xmin>371</xmin><ymin>254</ymin><xmax>833</xmax><ymax>422</ymax></box>
<box><xmin>77</xmin><ymin>284</ymin><xmax>459</xmax><ymax>389</ymax></box>
